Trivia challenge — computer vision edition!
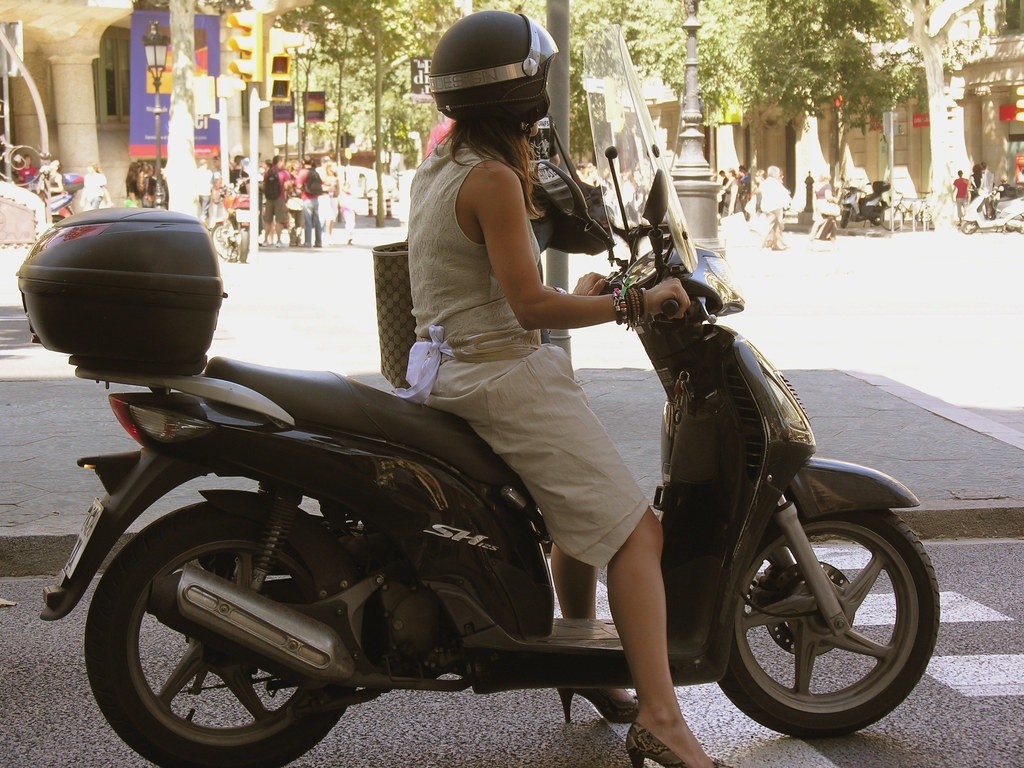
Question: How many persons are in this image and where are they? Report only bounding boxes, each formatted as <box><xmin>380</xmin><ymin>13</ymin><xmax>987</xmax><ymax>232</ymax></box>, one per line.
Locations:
<box><xmin>260</xmin><ymin>156</ymin><xmax>358</xmax><ymax>252</ymax></box>
<box><xmin>576</xmin><ymin>158</ymin><xmax>653</xmax><ymax>227</ymax></box>
<box><xmin>126</xmin><ymin>161</ymin><xmax>168</xmax><ymax>211</ymax></box>
<box><xmin>82</xmin><ymin>163</ymin><xmax>109</xmax><ymax>212</ymax></box>
<box><xmin>949</xmin><ymin>162</ymin><xmax>996</xmax><ymax>227</ymax></box>
<box><xmin>407</xmin><ymin>10</ymin><xmax>735</xmax><ymax>768</ymax></box>
<box><xmin>715</xmin><ymin>165</ymin><xmax>790</xmax><ymax>252</ymax></box>
<box><xmin>196</xmin><ymin>156</ymin><xmax>250</xmax><ymax>229</ymax></box>
<box><xmin>14</xmin><ymin>154</ymin><xmax>65</xmax><ymax>229</ymax></box>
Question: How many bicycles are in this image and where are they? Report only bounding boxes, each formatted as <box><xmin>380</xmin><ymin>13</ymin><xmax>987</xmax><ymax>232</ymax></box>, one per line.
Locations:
<box><xmin>880</xmin><ymin>191</ymin><xmax>936</xmax><ymax>231</ymax></box>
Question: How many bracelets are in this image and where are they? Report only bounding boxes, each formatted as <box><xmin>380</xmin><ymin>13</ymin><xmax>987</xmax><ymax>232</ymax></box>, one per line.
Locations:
<box><xmin>612</xmin><ymin>287</ymin><xmax>648</xmax><ymax>328</ymax></box>
<box><xmin>553</xmin><ymin>286</ymin><xmax>567</xmax><ymax>295</ymax></box>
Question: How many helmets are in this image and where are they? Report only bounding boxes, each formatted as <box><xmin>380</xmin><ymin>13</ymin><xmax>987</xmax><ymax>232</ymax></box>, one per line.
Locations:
<box><xmin>430</xmin><ymin>10</ymin><xmax>556</xmax><ymax>125</ymax></box>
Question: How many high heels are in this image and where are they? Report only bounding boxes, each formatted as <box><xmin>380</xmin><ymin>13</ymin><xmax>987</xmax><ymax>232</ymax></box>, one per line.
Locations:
<box><xmin>556</xmin><ymin>686</ymin><xmax>640</xmax><ymax>724</ymax></box>
<box><xmin>626</xmin><ymin>721</ymin><xmax>732</xmax><ymax>768</ymax></box>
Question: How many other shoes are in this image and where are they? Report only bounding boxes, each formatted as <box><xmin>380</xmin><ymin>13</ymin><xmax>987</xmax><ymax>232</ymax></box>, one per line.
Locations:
<box><xmin>262</xmin><ymin>237</ymin><xmax>323</xmax><ymax>248</ymax></box>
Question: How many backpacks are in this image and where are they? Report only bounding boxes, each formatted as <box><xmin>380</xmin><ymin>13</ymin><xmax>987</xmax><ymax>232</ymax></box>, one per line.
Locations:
<box><xmin>264</xmin><ymin>169</ymin><xmax>282</xmax><ymax>199</ymax></box>
<box><xmin>304</xmin><ymin>168</ymin><xmax>322</xmax><ymax>194</ymax></box>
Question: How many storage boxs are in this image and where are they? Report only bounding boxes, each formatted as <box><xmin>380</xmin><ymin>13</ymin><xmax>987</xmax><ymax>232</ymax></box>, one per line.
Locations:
<box><xmin>16</xmin><ymin>207</ymin><xmax>225</xmax><ymax>378</ymax></box>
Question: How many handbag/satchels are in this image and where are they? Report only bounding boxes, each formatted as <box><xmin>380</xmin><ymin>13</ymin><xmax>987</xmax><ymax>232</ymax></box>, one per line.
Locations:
<box><xmin>373</xmin><ymin>241</ymin><xmax>416</xmax><ymax>389</ymax></box>
<box><xmin>285</xmin><ymin>189</ymin><xmax>303</xmax><ymax>211</ymax></box>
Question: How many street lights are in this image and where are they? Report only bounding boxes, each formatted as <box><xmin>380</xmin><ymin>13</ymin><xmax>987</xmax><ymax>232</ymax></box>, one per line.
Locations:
<box><xmin>141</xmin><ymin>18</ymin><xmax>171</xmax><ymax>210</ymax></box>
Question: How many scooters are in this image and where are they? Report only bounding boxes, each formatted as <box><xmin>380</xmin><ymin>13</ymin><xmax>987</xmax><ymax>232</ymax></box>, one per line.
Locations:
<box><xmin>840</xmin><ymin>176</ymin><xmax>892</xmax><ymax>230</ymax></box>
<box><xmin>14</xmin><ymin>24</ymin><xmax>942</xmax><ymax>768</ymax></box>
<box><xmin>961</xmin><ymin>177</ymin><xmax>1024</xmax><ymax>235</ymax></box>
<box><xmin>20</xmin><ymin>171</ymin><xmax>84</xmax><ymax>224</ymax></box>
<box><xmin>210</xmin><ymin>179</ymin><xmax>251</xmax><ymax>262</ymax></box>
